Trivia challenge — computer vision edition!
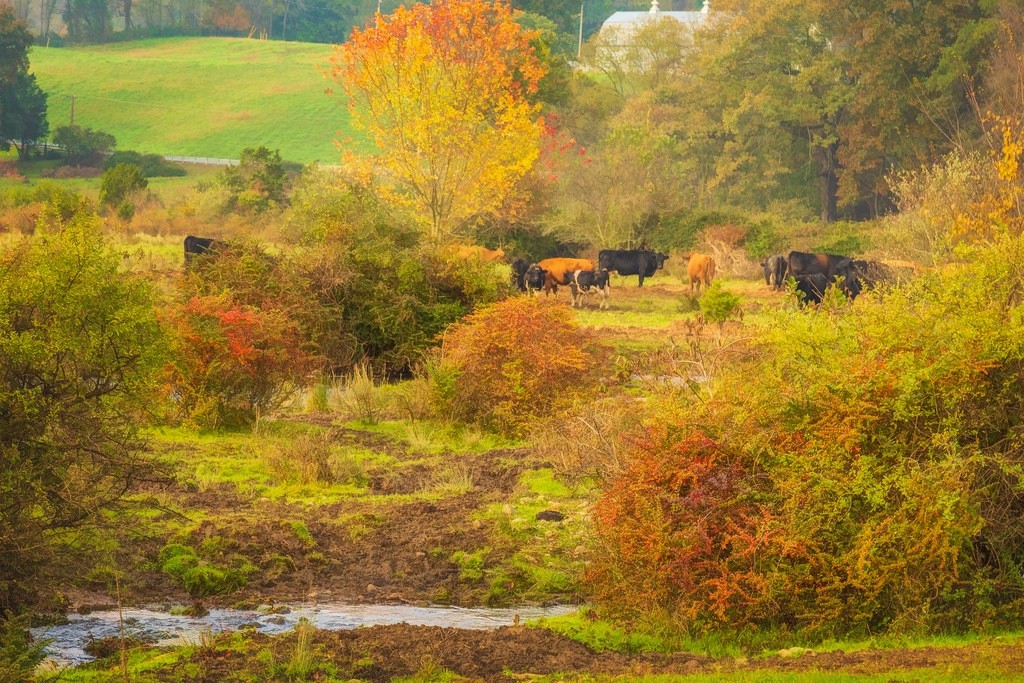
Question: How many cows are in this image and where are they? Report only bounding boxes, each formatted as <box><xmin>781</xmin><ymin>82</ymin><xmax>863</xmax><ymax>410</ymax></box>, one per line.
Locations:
<box><xmin>788</xmin><ymin>252</ymin><xmax>891</xmax><ymax>306</ymax></box>
<box><xmin>682</xmin><ymin>251</ymin><xmax>716</xmax><ymax>293</ymax></box>
<box><xmin>513</xmin><ymin>251</ymin><xmax>594</xmax><ymax>298</ymax></box>
<box><xmin>563</xmin><ymin>267</ymin><xmax>611</xmax><ymax>311</ymax></box>
<box><xmin>598</xmin><ymin>250</ymin><xmax>671</xmax><ymax>288</ymax></box>
<box><xmin>760</xmin><ymin>254</ymin><xmax>788</xmax><ymax>289</ymax></box>
